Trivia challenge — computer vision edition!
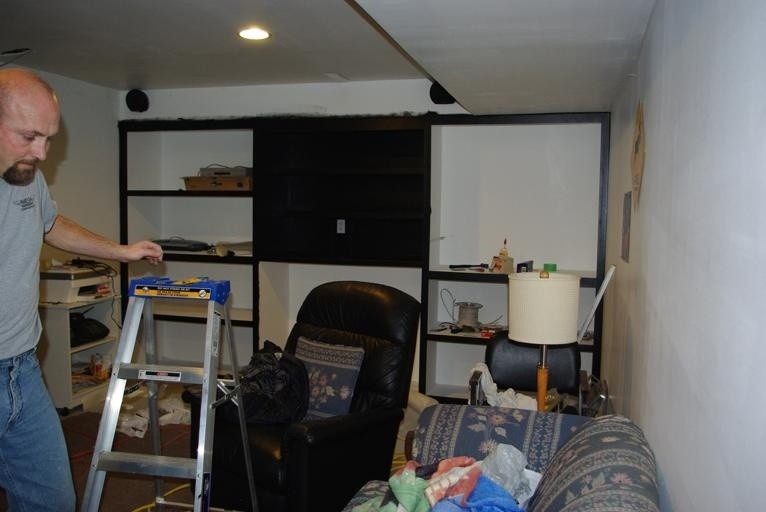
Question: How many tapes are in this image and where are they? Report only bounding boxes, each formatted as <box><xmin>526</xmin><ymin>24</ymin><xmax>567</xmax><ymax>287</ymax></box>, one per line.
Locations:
<box><xmin>543</xmin><ymin>263</ymin><xmax>557</xmax><ymax>273</ymax></box>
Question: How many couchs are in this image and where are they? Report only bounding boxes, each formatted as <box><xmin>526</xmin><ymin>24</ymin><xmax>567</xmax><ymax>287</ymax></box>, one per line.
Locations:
<box><xmin>344</xmin><ymin>404</ymin><xmax>662</xmax><ymax>512</ymax></box>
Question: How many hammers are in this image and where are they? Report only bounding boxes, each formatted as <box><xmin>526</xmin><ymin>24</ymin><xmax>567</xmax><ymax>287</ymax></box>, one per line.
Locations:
<box><xmin>448</xmin><ymin>262</ymin><xmax>489</xmax><ymax>270</ymax></box>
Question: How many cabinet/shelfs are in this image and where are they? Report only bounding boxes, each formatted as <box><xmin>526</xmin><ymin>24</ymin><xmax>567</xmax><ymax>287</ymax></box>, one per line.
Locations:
<box><xmin>253</xmin><ymin>113</ymin><xmax>427</xmax><ymax>268</ymax></box>
<box><xmin>118</xmin><ymin>118</ymin><xmax>259</xmax><ymax>374</ymax></box>
<box><xmin>418</xmin><ymin>109</ymin><xmax>611</xmax><ymax>405</ymax></box>
<box><xmin>38</xmin><ymin>293</ymin><xmax>123</xmax><ymax>413</ymax></box>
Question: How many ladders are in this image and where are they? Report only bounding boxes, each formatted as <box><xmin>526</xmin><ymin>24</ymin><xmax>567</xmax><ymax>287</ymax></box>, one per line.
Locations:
<box><xmin>78</xmin><ymin>276</ymin><xmax>262</xmax><ymax>512</ymax></box>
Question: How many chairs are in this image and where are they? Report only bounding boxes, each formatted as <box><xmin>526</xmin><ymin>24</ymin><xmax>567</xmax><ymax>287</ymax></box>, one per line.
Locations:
<box><xmin>468</xmin><ymin>331</ymin><xmax>581</xmax><ymax>412</ymax></box>
<box><xmin>181</xmin><ymin>280</ymin><xmax>421</xmax><ymax>512</ymax></box>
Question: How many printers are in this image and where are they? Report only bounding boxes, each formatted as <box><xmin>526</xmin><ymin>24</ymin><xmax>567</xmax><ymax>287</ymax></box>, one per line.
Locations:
<box><xmin>38</xmin><ymin>265</ymin><xmax>122</xmax><ymax>303</ymax></box>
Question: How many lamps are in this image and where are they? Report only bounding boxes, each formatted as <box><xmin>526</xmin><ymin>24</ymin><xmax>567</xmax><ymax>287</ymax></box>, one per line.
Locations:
<box><xmin>508</xmin><ymin>271</ymin><xmax>580</xmax><ymax>413</ymax></box>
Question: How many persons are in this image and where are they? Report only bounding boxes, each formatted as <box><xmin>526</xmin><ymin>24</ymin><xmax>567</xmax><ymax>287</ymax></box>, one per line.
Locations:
<box><xmin>0</xmin><ymin>67</ymin><xmax>164</xmax><ymax>512</ymax></box>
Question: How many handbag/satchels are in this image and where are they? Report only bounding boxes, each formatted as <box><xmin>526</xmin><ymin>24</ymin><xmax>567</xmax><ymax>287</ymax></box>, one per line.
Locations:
<box><xmin>240</xmin><ymin>353</ymin><xmax>309</xmax><ymax>426</ymax></box>
